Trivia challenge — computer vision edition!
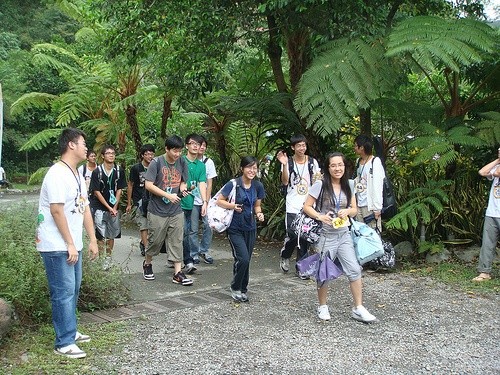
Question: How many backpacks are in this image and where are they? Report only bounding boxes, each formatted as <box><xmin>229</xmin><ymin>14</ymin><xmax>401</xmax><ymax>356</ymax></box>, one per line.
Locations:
<box><xmin>280</xmin><ymin>155</ymin><xmax>313</xmax><ymax>199</ymax></box>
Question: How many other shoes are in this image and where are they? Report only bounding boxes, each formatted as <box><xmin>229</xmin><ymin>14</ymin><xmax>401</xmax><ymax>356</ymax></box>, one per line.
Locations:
<box><xmin>473</xmin><ymin>274</ymin><xmax>491</xmax><ymax>281</ymax></box>
<box><xmin>366</xmin><ymin>267</ymin><xmax>380</xmax><ymax>272</ymax></box>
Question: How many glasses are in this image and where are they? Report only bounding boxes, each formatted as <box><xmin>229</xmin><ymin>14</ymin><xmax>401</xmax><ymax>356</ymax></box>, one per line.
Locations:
<box><xmin>145</xmin><ymin>153</ymin><xmax>155</xmax><ymax>156</ymax></box>
<box><xmin>187</xmin><ymin>141</ymin><xmax>201</xmax><ymax>146</ymax></box>
<box><xmin>105</xmin><ymin>151</ymin><xmax>114</xmax><ymax>155</ymax></box>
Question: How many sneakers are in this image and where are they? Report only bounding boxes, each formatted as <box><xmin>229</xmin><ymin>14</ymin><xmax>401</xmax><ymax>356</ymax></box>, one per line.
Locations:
<box><xmin>139</xmin><ymin>241</ymin><xmax>213</xmax><ymax>273</ymax></box>
<box><xmin>352</xmin><ymin>306</ymin><xmax>376</xmax><ymax>322</ymax></box>
<box><xmin>172</xmin><ymin>271</ymin><xmax>194</xmax><ymax>285</ymax></box>
<box><xmin>100</xmin><ymin>257</ymin><xmax>113</xmax><ymax>271</ymax></box>
<box><xmin>279</xmin><ymin>251</ymin><xmax>290</xmax><ymax>273</ymax></box>
<box><xmin>294</xmin><ymin>270</ymin><xmax>310</xmax><ymax>279</ymax></box>
<box><xmin>54</xmin><ymin>333</ymin><xmax>90</xmax><ymax>358</ymax></box>
<box><xmin>229</xmin><ymin>287</ymin><xmax>248</xmax><ymax>302</ymax></box>
<box><xmin>316</xmin><ymin>304</ymin><xmax>330</xmax><ymax>320</ymax></box>
<box><xmin>141</xmin><ymin>259</ymin><xmax>155</xmax><ymax>280</ymax></box>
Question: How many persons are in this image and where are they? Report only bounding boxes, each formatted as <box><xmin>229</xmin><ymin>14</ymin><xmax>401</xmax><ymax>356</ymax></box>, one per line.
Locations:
<box><xmin>166</xmin><ymin>132</ymin><xmax>207</xmax><ymax>272</ymax></box>
<box><xmin>277</xmin><ymin>134</ymin><xmax>323</xmax><ymax>280</ymax></box>
<box><xmin>303</xmin><ymin>152</ymin><xmax>377</xmax><ymax>321</ymax></box>
<box><xmin>0</xmin><ymin>166</ymin><xmax>7</xmax><ymax>199</ymax></box>
<box><xmin>188</xmin><ymin>135</ymin><xmax>217</xmax><ymax>263</ymax></box>
<box><xmin>352</xmin><ymin>135</ymin><xmax>385</xmax><ymax>271</ymax></box>
<box><xmin>471</xmin><ymin>145</ymin><xmax>500</xmax><ymax>284</ymax></box>
<box><xmin>37</xmin><ymin>126</ymin><xmax>92</xmax><ymax>357</ymax></box>
<box><xmin>76</xmin><ymin>148</ymin><xmax>99</xmax><ymax>203</ymax></box>
<box><xmin>90</xmin><ymin>145</ymin><xmax>127</xmax><ymax>270</ymax></box>
<box><xmin>142</xmin><ymin>134</ymin><xmax>194</xmax><ymax>285</ymax></box>
<box><xmin>128</xmin><ymin>143</ymin><xmax>155</xmax><ymax>255</ymax></box>
<box><xmin>215</xmin><ymin>154</ymin><xmax>265</xmax><ymax>302</ymax></box>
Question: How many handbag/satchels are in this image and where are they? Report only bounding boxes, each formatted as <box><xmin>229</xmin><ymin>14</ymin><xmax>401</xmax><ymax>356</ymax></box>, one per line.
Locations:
<box><xmin>207</xmin><ymin>179</ymin><xmax>236</xmax><ymax>233</ymax></box>
<box><xmin>371</xmin><ymin>157</ymin><xmax>397</xmax><ymax>221</ymax></box>
<box><xmin>352</xmin><ymin>220</ymin><xmax>384</xmax><ymax>265</ymax></box>
<box><xmin>368</xmin><ymin>220</ymin><xmax>395</xmax><ymax>268</ymax></box>
<box><xmin>290</xmin><ymin>179</ymin><xmax>324</xmax><ymax>244</ymax></box>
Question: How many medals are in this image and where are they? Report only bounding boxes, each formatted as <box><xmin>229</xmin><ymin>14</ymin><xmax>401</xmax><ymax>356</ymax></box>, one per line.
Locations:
<box><xmin>355</xmin><ymin>182</ymin><xmax>365</xmax><ymax>193</ymax></box>
<box><xmin>297</xmin><ymin>184</ymin><xmax>308</xmax><ymax>195</ymax></box>
<box><xmin>78</xmin><ymin>199</ymin><xmax>86</xmax><ymax>214</ymax></box>
<box><xmin>494</xmin><ymin>186</ymin><xmax>500</xmax><ymax>198</ymax></box>
